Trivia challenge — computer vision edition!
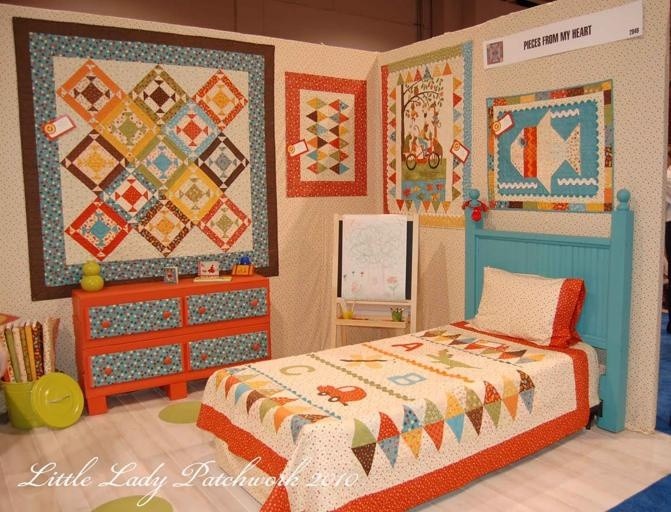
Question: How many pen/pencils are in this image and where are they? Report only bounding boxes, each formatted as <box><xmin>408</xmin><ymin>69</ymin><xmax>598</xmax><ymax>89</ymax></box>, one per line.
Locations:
<box><xmin>338</xmin><ymin>297</ymin><xmax>355</xmax><ymax>312</ymax></box>
<box><xmin>390</xmin><ymin>307</ymin><xmax>404</xmax><ymax>313</ymax></box>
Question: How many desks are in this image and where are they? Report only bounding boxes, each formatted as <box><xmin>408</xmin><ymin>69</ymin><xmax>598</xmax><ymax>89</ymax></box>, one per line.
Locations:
<box><xmin>72</xmin><ymin>271</ymin><xmax>272</xmax><ymax>417</ymax></box>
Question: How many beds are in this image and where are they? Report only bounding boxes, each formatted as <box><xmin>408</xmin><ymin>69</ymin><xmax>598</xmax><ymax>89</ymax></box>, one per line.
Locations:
<box><xmin>194</xmin><ymin>188</ymin><xmax>635</xmax><ymax>512</ymax></box>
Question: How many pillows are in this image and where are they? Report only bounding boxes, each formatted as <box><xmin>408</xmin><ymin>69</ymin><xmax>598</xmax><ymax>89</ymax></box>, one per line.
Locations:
<box><xmin>464</xmin><ymin>266</ymin><xmax>585</xmax><ymax>348</ymax></box>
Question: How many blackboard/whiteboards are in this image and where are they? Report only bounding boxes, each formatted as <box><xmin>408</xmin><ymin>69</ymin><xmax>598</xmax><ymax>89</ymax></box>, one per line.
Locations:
<box><xmin>332</xmin><ymin>213</ymin><xmax>420</xmax><ymax>306</ymax></box>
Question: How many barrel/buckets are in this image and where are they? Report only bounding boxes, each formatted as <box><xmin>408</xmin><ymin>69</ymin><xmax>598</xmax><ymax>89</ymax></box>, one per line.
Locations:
<box><xmin>0</xmin><ymin>368</ymin><xmax>65</xmax><ymax>430</ymax></box>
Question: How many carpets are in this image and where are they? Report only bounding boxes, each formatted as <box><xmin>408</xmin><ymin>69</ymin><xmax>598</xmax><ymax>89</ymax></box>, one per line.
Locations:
<box><xmin>655</xmin><ymin>312</ymin><xmax>671</xmax><ymax>434</ymax></box>
<box><xmin>607</xmin><ymin>472</ymin><xmax>671</xmax><ymax>512</ymax></box>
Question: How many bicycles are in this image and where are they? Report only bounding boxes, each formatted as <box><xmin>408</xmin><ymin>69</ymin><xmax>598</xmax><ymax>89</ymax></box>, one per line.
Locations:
<box><xmin>406</xmin><ymin>140</ymin><xmax>441</xmax><ymax>171</ymax></box>
